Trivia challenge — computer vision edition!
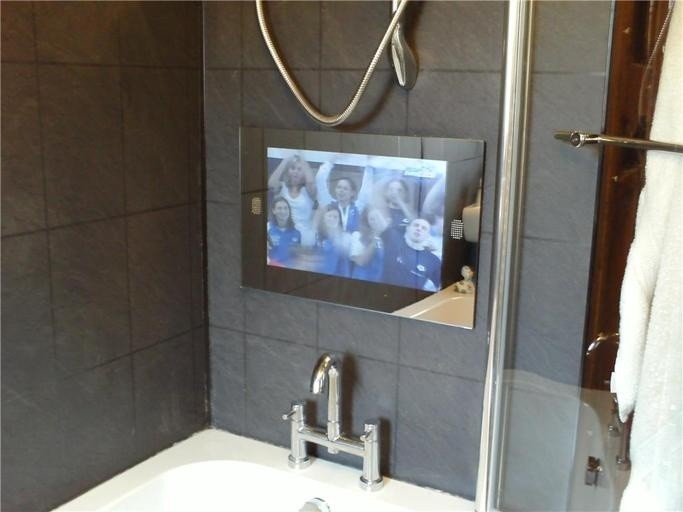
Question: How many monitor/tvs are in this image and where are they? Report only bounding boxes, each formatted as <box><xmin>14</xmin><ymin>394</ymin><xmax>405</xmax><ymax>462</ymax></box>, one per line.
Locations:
<box><xmin>238</xmin><ymin>127</ymin><xmax>486</xmax><ymax>330</ymax></box>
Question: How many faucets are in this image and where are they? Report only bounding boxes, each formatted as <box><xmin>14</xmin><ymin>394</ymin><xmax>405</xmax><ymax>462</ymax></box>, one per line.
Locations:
<box><xmin>584</xmin><ymin>328</ymin><xmax>621</xmax><ymax>439</ymax></box>
<box><xmin>282</xmin><ymin>352</ymin><xmax>384</xmax><ymax>493</ymax></box>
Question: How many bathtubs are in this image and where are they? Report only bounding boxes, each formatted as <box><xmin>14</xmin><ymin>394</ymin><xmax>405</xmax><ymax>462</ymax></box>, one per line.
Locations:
<box><xmin>49</xmin><ymin>425</ymin><xmax>476</xmax><ymax>512</ymax></box>
<box><xmin>497</xmin><ymin>368</ymin><xmax>631</xmax><ymax>512</ymax></box>
<box><xmin>390</xmin><ymin>279</ymin><xmax>476</xmax><ymax>330</ymax></box>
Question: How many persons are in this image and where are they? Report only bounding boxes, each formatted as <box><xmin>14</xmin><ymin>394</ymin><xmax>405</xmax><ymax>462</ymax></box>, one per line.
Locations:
<box><xmin>267</xmin><ymin>147</ymin><xmax>447</xmax><ymax>294</ymax></box>
